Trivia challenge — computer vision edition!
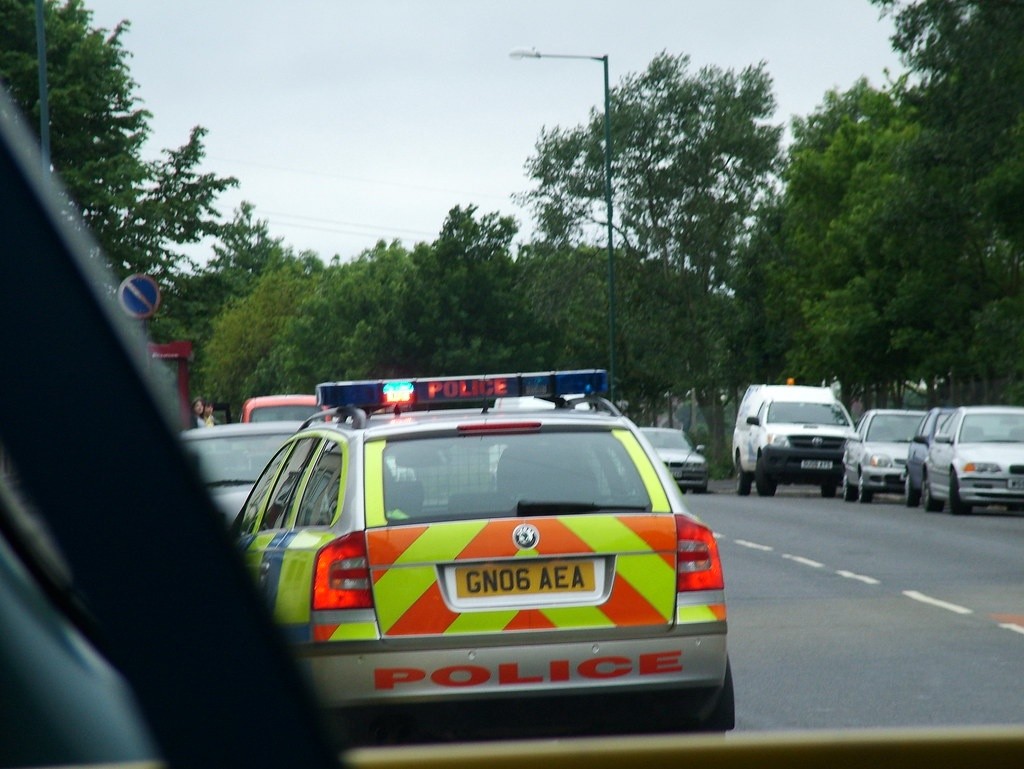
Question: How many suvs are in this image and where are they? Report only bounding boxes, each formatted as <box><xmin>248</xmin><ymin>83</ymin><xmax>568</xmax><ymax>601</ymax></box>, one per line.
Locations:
<box><xmin>732</xmin><ymin>384</ymin><xmax>856</xmax><ymax>500</ymax></box>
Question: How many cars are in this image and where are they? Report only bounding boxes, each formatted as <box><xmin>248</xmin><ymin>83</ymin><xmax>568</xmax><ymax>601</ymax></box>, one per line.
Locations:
<box><xmin>842</xmin><ymin>410</ymin><xmax>928</xmax><ymax>504</ymax></box>
<box><xmin>925</xmin><ymin>404</ymin><xmax>1024</xmax><ymax>513</ymax></box>
<box><xmin>902</xmin><ymin>408</ymin><xmax>955</xmax><ymax>508</ymax></box>
<box><xmin>227</xmin><ymin>365</ymin><xmax>736</xmax><ymax>746</ymax></box>
<box><xmin>175</xmin><ymin>420</ymin><xmax>321</xmax><ymax>534</ymax></box>
<box><xmin>638</xmin><ymin>426</ymin><xmax>709</xmax><ymax>495</ymax></box>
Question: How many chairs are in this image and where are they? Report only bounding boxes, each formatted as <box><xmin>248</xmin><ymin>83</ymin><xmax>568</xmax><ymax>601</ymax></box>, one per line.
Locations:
<box><xmin>378</xmin><ymin>480</ymin><xmax>424</xmax><ymax>521</ymax></box>
<box><xmin>497</xmin><ymin>439</ymin><xmax>601</xmax><ymax>511</ymax></box>
<box><xmin>964</xmin><ymin>424</ymin><xmax>985</xmax><ymax>442</ymax></box>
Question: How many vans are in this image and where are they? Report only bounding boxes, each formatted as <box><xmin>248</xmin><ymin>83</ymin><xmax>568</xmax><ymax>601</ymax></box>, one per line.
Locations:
<box><xmin>237</xmin><ymin>394</ymin><xmax>333</xmax><ymax>423</ymax></box>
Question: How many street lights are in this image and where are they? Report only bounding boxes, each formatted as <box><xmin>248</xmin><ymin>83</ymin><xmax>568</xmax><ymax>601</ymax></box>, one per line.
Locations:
<box><xmin>511</xmin><ymin>46</ymin><xmax>617</xmax><ymax>409</ymax></box>
